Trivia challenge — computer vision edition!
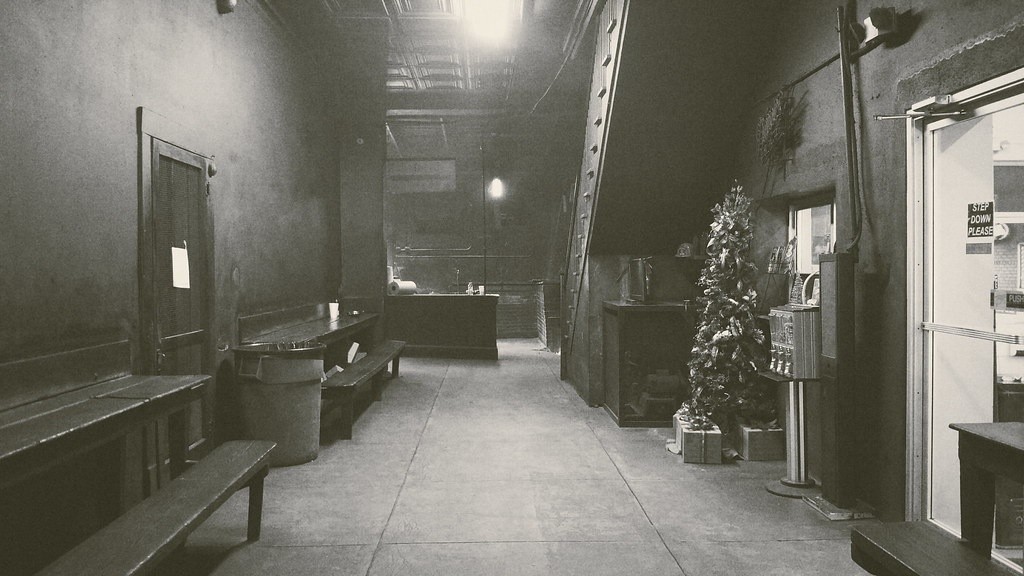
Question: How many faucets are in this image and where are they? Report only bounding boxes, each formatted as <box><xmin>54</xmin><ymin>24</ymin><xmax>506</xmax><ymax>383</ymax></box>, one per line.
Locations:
<box><xmin>455</xmin><ymin>269</ymin><xmax>460</xmax><ymax>292</ymax></box>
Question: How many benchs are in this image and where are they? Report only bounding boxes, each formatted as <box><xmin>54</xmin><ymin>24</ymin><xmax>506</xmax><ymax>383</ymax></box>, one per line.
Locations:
<box><xmin>850</xmin><ymin>519</ymin><xmax>1024</xmax><ymax>576</ymax></box>
<box><xmin>321</xmin><ymin>340</ymin><xmax>407</xmax><ymax>441</ymax></box>
<box><xmin>26</xmin><ymin>440</ymin><xmax>279</xmax><ymax>576</ymax></box>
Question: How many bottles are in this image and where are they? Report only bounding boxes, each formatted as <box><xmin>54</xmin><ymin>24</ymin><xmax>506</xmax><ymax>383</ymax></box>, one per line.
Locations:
<box><xmin>468</xmin><ymin>282</ymin><xmax>473</xmax><ymax>295</ymax></box>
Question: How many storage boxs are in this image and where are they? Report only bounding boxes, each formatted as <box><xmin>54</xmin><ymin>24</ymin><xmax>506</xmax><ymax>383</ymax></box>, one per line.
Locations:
<box><xmin>671</xmin><ymin>411</ymin><xmax>723</xmax><ymax>464</ymax></box>
<box><xmin>734</xmin><ymin>423</ymin><xmax>786</xmax><ymax>461</ymax></box>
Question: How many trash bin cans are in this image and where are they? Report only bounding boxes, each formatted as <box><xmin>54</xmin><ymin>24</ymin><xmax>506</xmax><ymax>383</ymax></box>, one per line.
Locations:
<box><xmin>234</xmin><ymin>341</ymin><xmax>321</xmax><ymax>468</ymax></box>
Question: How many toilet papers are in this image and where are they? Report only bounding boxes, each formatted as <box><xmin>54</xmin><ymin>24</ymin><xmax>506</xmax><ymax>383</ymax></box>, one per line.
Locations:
<box><xmin>387</xmin><ymin>265</ymin><xmax>416</xmax><ymax>295</ymax></box>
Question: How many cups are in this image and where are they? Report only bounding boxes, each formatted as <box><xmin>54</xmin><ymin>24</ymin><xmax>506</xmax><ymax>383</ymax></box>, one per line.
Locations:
<box><xmin>328</xmin><ymin>302</ymin><xmax>340</xmax><ymax>319</ymax></box>
<box><xmin>479</xmin><ymin>286</ymin><xmax>484</xmax><ymax>295</ymax></box>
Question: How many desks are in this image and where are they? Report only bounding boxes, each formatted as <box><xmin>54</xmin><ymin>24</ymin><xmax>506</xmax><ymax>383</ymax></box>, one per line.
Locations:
<box><xmin>948</xmin><ymin>422</ymin><xmax>1024</xmax><ymax>558</ymax></box>
<box><xmin>0</xmin><ymin>372</ymin><xmax>220</xmax><ymax>482</ymax></box>
<box><xmin>241</xmin><ymin>310</ymin><xmax>378</xmax><ymax>353</ymax></box>
